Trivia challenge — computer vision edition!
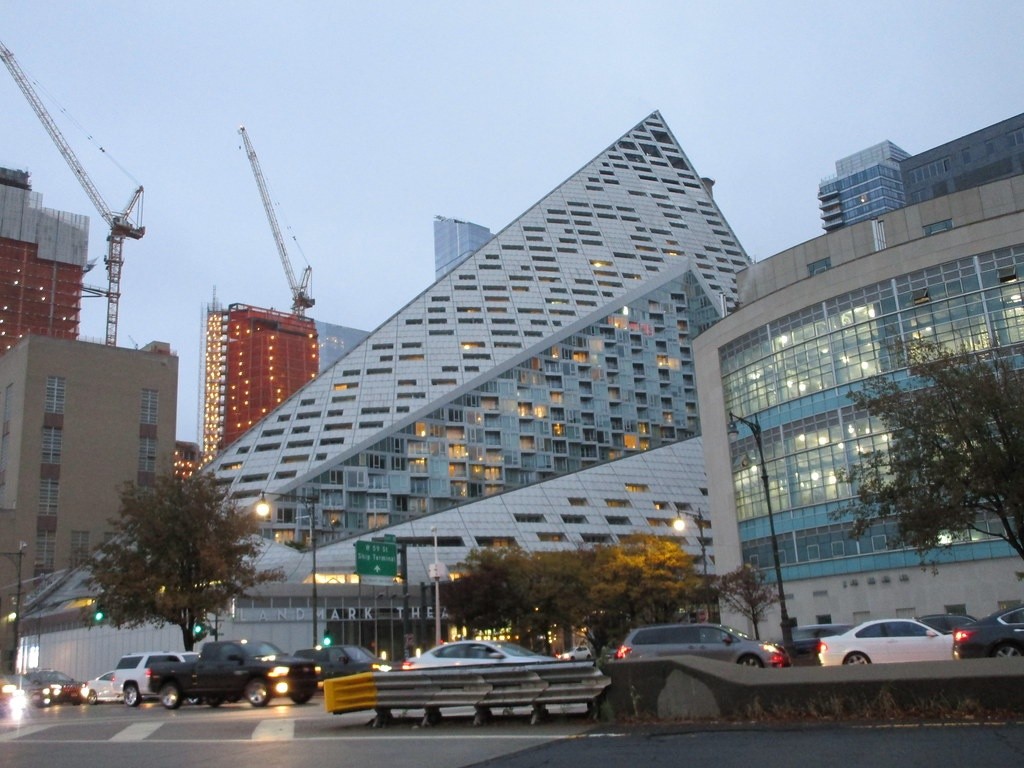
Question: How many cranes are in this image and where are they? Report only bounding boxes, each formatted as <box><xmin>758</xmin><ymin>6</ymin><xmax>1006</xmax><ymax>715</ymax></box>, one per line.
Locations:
<box><xmin>237</xmin><ymin>125</ymin><xmax>318</xmax><ymax>312</ymax></box>
<box><xmin>0</xmin><ymin>40</ymin><xmax>146</xmax><ymax>346</ymax></box>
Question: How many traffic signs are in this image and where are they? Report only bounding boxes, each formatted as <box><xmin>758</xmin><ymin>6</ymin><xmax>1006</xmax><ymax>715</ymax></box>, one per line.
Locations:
<box><xmin>354</xmin><ymin>541</ymin><xmax>398</xmax><ymax>576</ymax></box>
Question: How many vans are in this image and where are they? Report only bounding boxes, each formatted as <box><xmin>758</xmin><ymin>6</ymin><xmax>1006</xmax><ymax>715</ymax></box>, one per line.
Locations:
<box><xmin>617</xmin><ymin>622</ymin><xmax>794</xmax><ymax>670</ymax></box>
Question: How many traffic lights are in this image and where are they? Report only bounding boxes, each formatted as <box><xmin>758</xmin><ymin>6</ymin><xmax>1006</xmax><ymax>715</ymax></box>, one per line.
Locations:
<box><xmin>195</xmin><ymin>622</ymin><xmax>203</xmax><ymax>639</ymax></box>
<box><xmin>94</xmin><ymin>595</ymin><xmax>106</xmax><ymax>621</ymax></box>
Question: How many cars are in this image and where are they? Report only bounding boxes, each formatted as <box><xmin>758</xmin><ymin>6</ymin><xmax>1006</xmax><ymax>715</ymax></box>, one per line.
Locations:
<box><xmin>792</xmin><ymin>625</ymin><xmax>858</xmax><ymax>667</ymax></box>
<box><xmin>0</xmin><ymin>667</ymin><xmax>85</xmax><ymax>715</ymax></box>
<box><xmin>402</xmin><ymin>638</ymin><xmax>561</xmax><ymax>666</ymax></box>
<box><xmin>917</xmin><ymin>614</ymin><xmax>976</xmax><ymax>634</ymax></box>
<box><xmin>297</xmin><ymin>644</ymin><xmax>393</xmax><ymax>678</ymax></box>
<box><xmin>818</xmin><ymin>617</ymin><xmax>954</xmax><ymax>666</ymax></box>
<box><xmin>953</xmin><ymin>605</ymin><xmax>1024</xmax><ymax>659</ymax></box>
<box><xmin>83</xmin><ymin>672</ymin><xmax>124</xmax><ymax>704</ymax></box>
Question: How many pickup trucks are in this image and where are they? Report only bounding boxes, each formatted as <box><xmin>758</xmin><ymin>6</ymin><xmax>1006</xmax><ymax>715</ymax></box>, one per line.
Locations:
<box><xmin>147</xmin><ymin>637</ymin><xmax>322</xmax><ymax>704</ymax></box>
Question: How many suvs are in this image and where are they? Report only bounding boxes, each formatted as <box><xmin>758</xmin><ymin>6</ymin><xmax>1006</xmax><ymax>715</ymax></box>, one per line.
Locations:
<box><xmin>113</xmin><ymin>650</ymin><xmax>203</xmax><ymax>704</ymax></box>
<box><xmin>556</xmin><ymin>646</ymin><xmax>593</xmax><ymax>660</ymax></box>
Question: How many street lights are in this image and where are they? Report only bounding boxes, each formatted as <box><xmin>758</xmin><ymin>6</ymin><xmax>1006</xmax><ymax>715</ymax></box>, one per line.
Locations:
<box><xmin>257</xmin><ymin>490</ymin><xmax>319</xmax><ymax>649</ymax></box>
<box><xmin>726</xmin><ymin>412</ymin><xmax>791</xmax><ymax>640</ymax></box>
<box><xmin>674</xmin><ymin>511</ymin><xmax>711</xmax><ymax>620</ymax></box>
